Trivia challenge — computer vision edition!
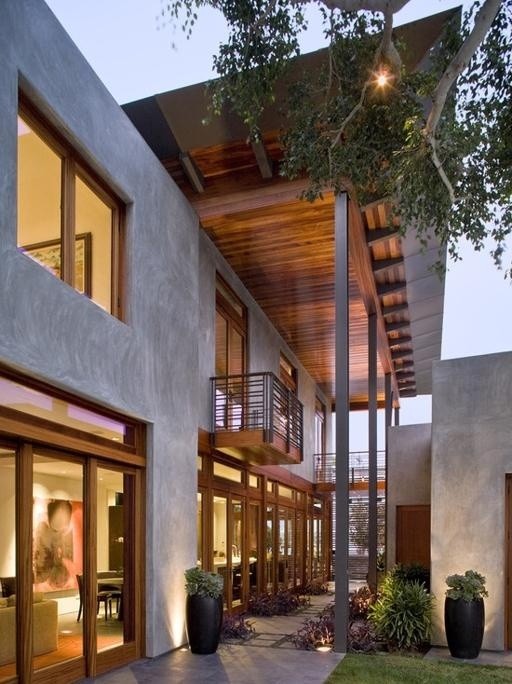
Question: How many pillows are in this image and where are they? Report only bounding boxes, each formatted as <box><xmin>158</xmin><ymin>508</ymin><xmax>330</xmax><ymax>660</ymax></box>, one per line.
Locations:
<box><xmin>6</xmin><ymin>591</ymin><xmax>42</xmax><ymax>607</ymax></box>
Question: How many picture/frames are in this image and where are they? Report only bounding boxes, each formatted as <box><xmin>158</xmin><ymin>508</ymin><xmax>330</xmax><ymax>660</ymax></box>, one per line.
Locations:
<box><xmin>17</xmin><ymin>232</ymin><xmax>93</xmax><ymax>300</ymax></box>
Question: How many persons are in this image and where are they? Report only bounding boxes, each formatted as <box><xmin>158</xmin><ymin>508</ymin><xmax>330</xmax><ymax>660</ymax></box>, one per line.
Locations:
<box><xmin>32</xmin><ymin>500</ymin><xmax>74</xmax><ymax>588</ymax></box>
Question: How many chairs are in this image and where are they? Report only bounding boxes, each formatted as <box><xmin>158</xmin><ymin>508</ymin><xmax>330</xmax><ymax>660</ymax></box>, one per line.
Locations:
<box><xmin>0</xmin><ymin>597</ymin><xmax>59</xmax><ymax>667</ymax></box>
<box><xmin>74</xmin><ymin>571</ymin><xmax>124</xmax><ymax>623</ymax></box>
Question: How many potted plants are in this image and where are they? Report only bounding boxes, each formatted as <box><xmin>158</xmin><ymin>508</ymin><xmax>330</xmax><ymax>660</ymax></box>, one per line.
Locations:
<box><xmin>444</xmin><ymin>570</ymin><xmax>488</xmax><ymax>659</ymax></box>
<box><xmin>184</xmin><ymin>567</ymin><xmax>224</xmax><ymax>655</ymax></box>
